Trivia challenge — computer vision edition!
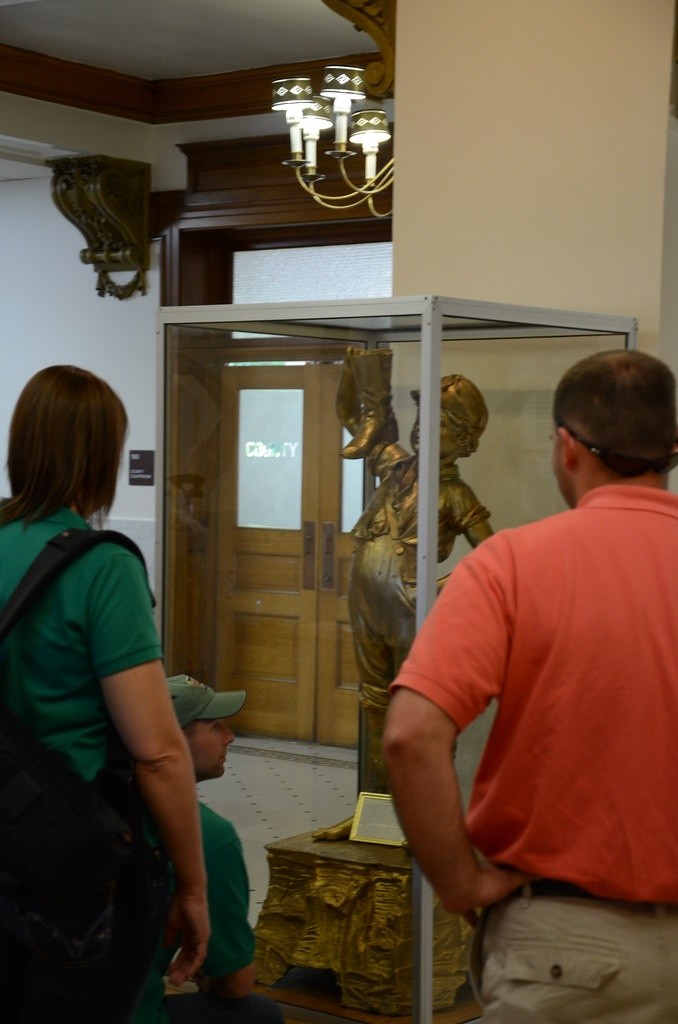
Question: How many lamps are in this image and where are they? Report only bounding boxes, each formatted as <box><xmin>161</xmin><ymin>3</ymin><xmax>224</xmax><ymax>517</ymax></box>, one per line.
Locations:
<box><xmin>270</xmin><ymin>63</ymin><xmax>395</xmax><ymax>218</ymax></box>
<box><xmin>41</xmin><ymin>154</ymin><xmax>151</xmax><ymax>300</ymax></box>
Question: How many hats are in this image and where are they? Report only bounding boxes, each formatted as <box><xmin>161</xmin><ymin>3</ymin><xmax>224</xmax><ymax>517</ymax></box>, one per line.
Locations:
<box><xmin>166</xmin><ymin>674</ymin><xmax>246</xmax><ymax>728</ymax></box>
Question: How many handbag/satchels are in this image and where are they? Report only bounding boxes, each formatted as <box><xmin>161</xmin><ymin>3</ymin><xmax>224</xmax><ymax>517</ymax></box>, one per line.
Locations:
<box><xmin>1</xmin><ymin>529</ymin><xmax>157</xmax><ymax>932</ymax></box>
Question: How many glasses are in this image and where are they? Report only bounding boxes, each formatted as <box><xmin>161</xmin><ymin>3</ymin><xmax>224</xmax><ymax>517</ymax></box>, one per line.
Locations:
<box><xmin>561</xmin><ymin>423</ymin><xmax>678</xmax><ymax>478</ymax></box>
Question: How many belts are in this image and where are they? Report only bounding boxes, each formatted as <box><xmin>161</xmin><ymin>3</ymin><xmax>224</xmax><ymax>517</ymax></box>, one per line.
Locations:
<box><xmin>512</xmin><ymin>877</ymin><xmax>595</xmax><ymax>899</ymax></box>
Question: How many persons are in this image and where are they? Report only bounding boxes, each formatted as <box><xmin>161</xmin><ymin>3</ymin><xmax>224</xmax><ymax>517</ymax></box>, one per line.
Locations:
<box><xmin>1</xmin><ymin>364</ymin><xmax>212</xmax><ymax>1024</ymax></box>
<box><xmin>167</xmin><ymin>674</ymin><xmax>286</xmax><ymax>1024</ymax></box>
<box><xmin>310</xmin><ymin>346</ymin><xmax>495</xmax><ymax>840</ymax></box>
<box><xmin>380</xmin><ymin>346</ymin><xmax>677</xmax><ymax>1024</ymax></box>
